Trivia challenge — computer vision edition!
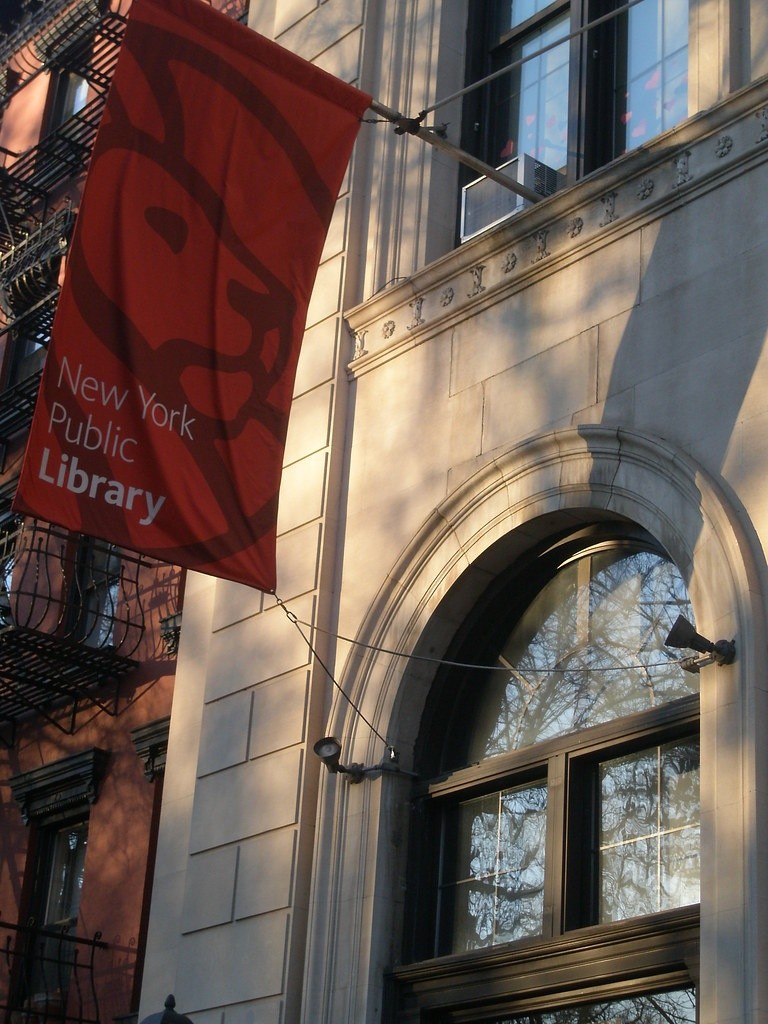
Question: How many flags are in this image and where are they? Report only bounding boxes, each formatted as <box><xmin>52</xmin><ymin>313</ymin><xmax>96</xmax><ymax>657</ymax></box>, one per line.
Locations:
<box><xmin>13</xmin><ymin>0</ymin><xmax>376</xmax><ymax>593</ymax></box>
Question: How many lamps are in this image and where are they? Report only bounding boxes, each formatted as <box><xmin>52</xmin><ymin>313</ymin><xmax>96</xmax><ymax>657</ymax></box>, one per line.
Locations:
<box><xmin>663</xmin><ymin>614</ymin><xmax>735</xmax><ymax>673</ymax></box>
<box><xmin>313</xmin><ymin>736</ymin><xmax>362</xmax><ymax>785</ymax></box>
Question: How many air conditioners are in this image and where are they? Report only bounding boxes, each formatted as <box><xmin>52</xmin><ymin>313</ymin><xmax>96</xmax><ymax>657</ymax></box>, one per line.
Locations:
<box><xmin>460</xmin><ymin>152</ymin><xmax>566</xmax><ymax>243</ymax></box>
